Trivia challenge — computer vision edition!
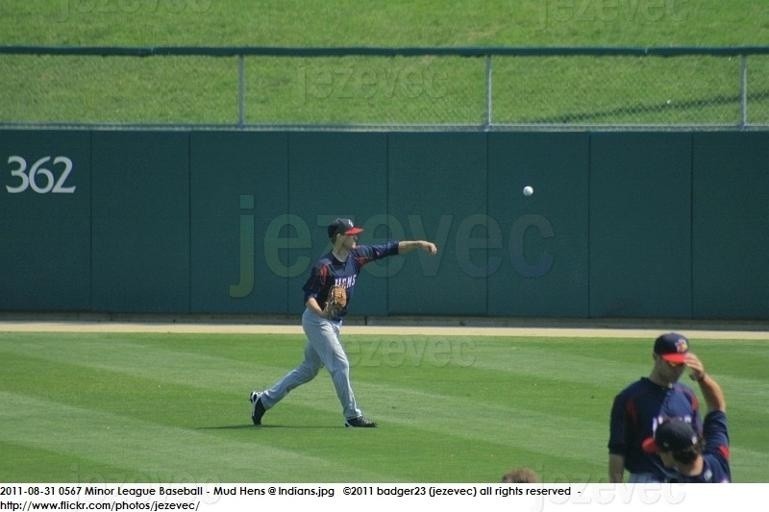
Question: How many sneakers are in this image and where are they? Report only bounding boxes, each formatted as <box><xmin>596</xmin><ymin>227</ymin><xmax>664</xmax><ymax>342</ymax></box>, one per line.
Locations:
<box><xmin>344</xmin><ymin>416</ymin><xmax>377</xmax><ymax>428</ymax></box>
<box><xmin>249</xmin><ymin>388</ymin><xmax>265</xmax><ymax>427</ymax></box>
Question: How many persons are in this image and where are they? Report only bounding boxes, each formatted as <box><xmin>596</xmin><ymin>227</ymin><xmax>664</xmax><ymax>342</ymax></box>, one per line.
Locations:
<box><xmin>642</xmin><ymin>350</ymin><xmax>733</xmax><ymax>483</ymax></box>
<box><xmin>501</xmin><ymin>468</ymin><xmax>539</xmax><ymax>483</ymax></box>
<box><xmin>608</xmin><ymin>333</ymin><xmax>704</xmax><ymax>484</ymax></box>
<box><xmin>250</xmin><ymin>217</ymin><xmax>437</xmax><ymax>428</ymax></box>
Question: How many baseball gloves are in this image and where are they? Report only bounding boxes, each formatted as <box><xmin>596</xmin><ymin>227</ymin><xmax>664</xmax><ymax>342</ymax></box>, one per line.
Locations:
<box><xmin>327</xmin><ymin>286</ymin><xmax>347</xmax><ymax>319</ymax></box>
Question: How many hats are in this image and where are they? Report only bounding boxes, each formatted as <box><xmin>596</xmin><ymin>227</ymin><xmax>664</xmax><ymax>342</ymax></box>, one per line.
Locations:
<box><xmin>651</xmin><ymin>332</ymin><xmax>691</xmax><ymax>365</ymax></box>
<box><xmin>327</xmin><ymin>217</ymin><xmax>365</xmax><ymax>237</ymax></box>
<box><xmin>640</xmin><ymin>417</ymin><xmax>698</xmax><ymax>454</ymax></box>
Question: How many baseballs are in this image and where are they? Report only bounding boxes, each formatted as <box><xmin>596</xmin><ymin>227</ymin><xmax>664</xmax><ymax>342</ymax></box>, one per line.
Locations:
<box><xmin>523</xmin><ymin>186</ymin><xmax>533</xmax><ymax>197</ymax></box>
